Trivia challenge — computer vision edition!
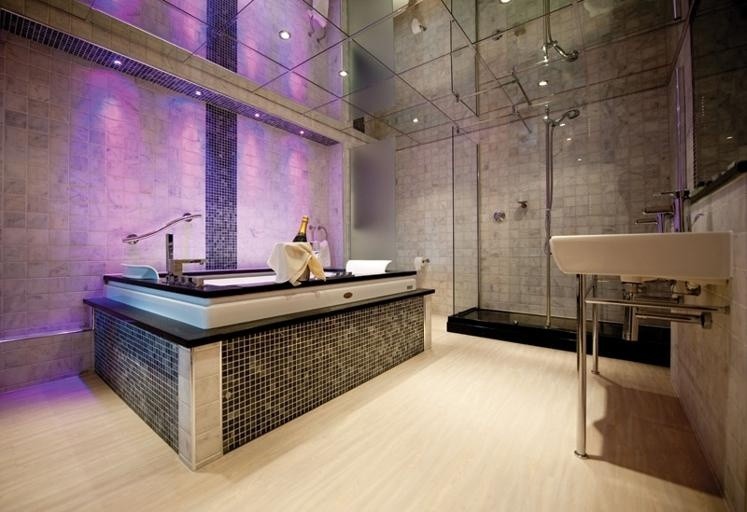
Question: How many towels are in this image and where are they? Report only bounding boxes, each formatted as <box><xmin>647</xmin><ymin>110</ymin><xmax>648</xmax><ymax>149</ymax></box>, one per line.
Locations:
<box><xmin>266</xmin><ymin>241</ymin><xmax>326</xmax><ymax>287</ymax></box>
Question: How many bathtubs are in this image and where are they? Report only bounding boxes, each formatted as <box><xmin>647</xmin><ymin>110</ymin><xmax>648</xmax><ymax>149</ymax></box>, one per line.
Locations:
<box><xmin>102</xmin><ymin>270</ymin><xmax>417</xmax><ymax>329</ymax></box>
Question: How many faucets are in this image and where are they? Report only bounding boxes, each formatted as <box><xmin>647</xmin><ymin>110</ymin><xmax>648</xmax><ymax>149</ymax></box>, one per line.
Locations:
<box><xmin>642</xmin><ymin>188</ymin><xmax>690</xmax><ymax>232</ymax></box>
<box><xmin>634</xmin><ymin>212</ymin><xmax>666</xmax><ymax>232</ymax></box>
<box><xmin>165</xmin><ymin>258</ymin><xmax>205</xmax><ymax>274</ymax></box>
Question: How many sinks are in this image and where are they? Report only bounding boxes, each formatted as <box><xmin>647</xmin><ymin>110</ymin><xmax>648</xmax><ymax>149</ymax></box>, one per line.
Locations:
<box><xmin>549</xmin><ymin>230</ymin><xmax>731</xmax><ymax>283</ymax></box>
